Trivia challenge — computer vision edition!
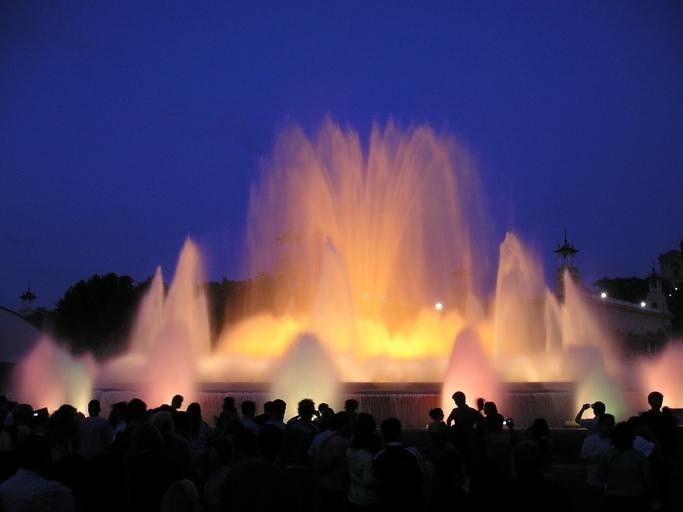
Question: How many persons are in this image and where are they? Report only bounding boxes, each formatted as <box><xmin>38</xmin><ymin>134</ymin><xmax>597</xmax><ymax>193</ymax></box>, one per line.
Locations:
<box><xmin>1</xmin><ymin>391</ymin><xmax>683</xmax><ymax>511</ymax></box>
